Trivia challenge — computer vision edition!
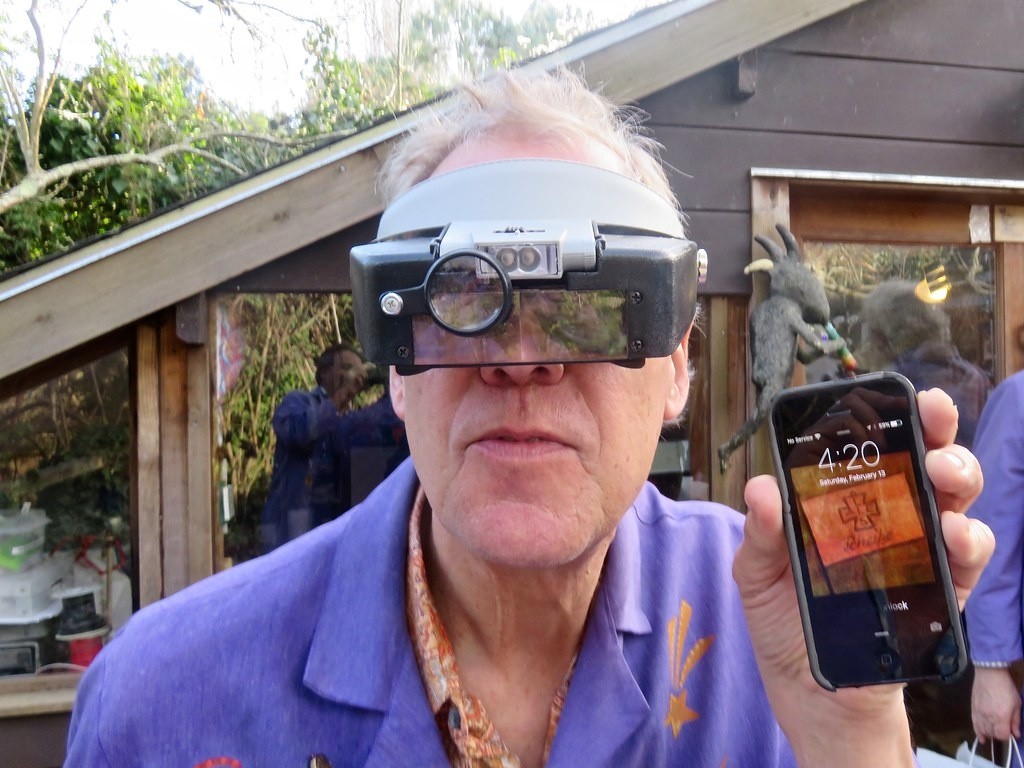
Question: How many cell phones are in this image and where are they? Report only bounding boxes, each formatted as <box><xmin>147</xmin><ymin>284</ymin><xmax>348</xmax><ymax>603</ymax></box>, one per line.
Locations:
<box><xmin>768</xmin><ymin>370</ymin><xmax>968</xmax><ymax>692</ymax></box>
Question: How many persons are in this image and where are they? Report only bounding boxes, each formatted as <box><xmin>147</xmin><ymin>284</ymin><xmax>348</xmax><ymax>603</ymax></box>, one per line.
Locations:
<box><xmin>786</xmin><ymin>385</ymin><xmax>907</xmax><ymax>468</ymax></box>
<box><xmin>861</xmin><ymin>282</ymin><xmax>993</xmax><ymax>450</ymax></box>
<box><xmin>964</xmin><ymin>370</ymin><xmax>1024</xmax><ymax>768</ymax></box>
<box><xmin>60</xmin><ymin>67</ymin><xmax>995</xmax><ymax>768</ymax></box>
<box><xmin>263</xmin><ymin>343</ymin><xmax>412</xmax><ymax>557</ymax></box>
<box><xmin>416</xmin><ymin>270</ymin><xmax>606</xmax><ymax>358</ymax></box>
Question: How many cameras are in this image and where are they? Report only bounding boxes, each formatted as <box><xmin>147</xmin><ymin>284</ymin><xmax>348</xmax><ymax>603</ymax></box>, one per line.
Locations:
<box><xmin>361</xmin><ymin>369</ymin><xmax>383</xmax><ymax>388</ymax></box>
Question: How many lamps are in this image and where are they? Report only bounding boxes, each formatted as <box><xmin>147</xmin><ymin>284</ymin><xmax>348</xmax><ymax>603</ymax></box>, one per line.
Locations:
<box><xmin>915</xmin><ymin>253</ymin><xmax>996</xmax><ymax>303</ymax></box>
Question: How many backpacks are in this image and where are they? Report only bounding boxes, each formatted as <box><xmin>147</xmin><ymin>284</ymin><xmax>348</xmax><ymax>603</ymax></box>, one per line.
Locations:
<box><xmin>225</xmin><ymin>469</ymin><xmax>288</xmax><ymax>565</ymax></box>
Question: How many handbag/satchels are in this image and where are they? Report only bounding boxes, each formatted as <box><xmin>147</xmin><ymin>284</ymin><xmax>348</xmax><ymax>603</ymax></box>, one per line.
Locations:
<box><xmin>915</xmin><ymin>728</ymin><xmax>1024</xmax><ymax>768</ymax></box>
